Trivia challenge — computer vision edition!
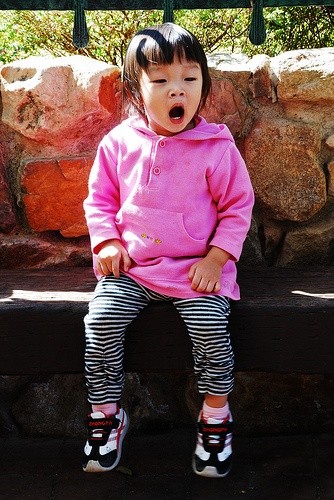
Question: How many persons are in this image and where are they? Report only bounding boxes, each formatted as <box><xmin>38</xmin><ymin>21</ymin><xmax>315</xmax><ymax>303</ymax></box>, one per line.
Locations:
<box><xmin>82</xmin><ymin>22</ymin><xmax>254</xmax><ymax>476</ymax></box>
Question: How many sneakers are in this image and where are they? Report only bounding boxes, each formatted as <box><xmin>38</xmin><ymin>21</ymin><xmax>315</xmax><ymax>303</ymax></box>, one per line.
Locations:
<box><xmin>82</xmin><ymin>408</ymin><xmax>130</xmax><ymax>472</ymax></box>
<box><xmin>193</xmin><ymin>410</ymin><xmax>233</xmax><ymax>477</ymax></box>
